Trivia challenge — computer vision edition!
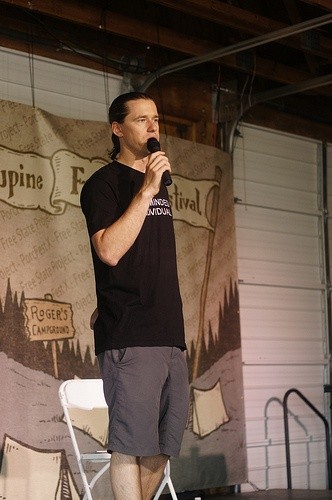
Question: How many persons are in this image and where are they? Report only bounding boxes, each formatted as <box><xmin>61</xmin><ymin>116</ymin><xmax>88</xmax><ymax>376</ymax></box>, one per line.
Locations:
<box><xmin>79</xmin><ymin>93</ymin><xmax>189</xmax><ymax>500</ymax></box>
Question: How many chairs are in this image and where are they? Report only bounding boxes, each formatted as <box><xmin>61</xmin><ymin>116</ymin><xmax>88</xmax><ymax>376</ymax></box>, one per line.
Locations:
<box><xmin>54</xmin><ymin>379</ymin><xmax>178</xmax><ymax>500</ymax></box>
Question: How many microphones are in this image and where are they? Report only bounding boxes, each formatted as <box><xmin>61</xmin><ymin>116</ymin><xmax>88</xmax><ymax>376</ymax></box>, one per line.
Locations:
<box><xmin>147</xmin><ymin>138</ymin><xmax>172</xmax><ymax>186</ymax></box>
